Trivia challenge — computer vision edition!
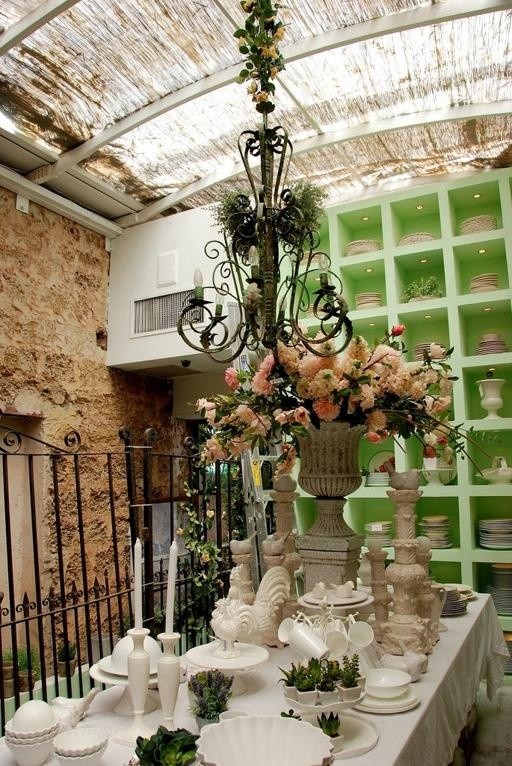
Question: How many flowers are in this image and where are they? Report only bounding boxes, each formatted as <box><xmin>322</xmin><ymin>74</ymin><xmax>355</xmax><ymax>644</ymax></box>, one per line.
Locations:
<box><xmin>232</xmin><ymin>0</ymin><xmax>292</xmax><ymax>103</ymax></box>
<box><xmin>187</xmin><ymin>320</ymin><xmax>504</xmax><ymax>478</ymax></box>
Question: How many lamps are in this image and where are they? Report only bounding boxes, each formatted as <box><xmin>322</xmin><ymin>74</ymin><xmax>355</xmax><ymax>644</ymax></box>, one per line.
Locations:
<box><xmin>170</xmin><ymin>0</ymin><xmax>357</xmax><ymax>365</ymax></box>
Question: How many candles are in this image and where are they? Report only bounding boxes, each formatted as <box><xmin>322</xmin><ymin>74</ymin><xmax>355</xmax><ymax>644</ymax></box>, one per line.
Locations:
<box><xmin>133</xmin><ymin>536</ymin><xmax>142</xmax><ymax>625</ymax></box>
<box><xmin>166</xmin><ymin>537</ymin><xmax>178</xmax><ymax>633</ymax></box>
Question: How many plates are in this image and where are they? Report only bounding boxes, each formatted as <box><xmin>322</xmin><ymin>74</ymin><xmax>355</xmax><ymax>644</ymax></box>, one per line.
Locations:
<box><xmin>368</xmin><ymin>449</ymin><xmax>394</xmax><ymax>472</ymax></box>
<box><xmin>400</xmin><ymin>232</ymin><xmax>433</xmax><ymax>245</ymax></box>
<box><xmin>353</xmin><ymin>688</ymin><xmax>421</xmax><ymax>708</ymax></box>
<box><xmin>307</xmin><ymin>304</ymin><xmax>323</xmax><ymax>316</ymax></box>
<box><xmin>185</xmin><ymin>642</ymin><xmax>269</xmax><ymax>668</ymax></box>
<box><xmin>346</xmin><ymin>240</ymin><xmax>379</xmax><ymax>255</ymax></box>
<box><xmin>417</xmin><ymin>516</ymin><xmax>454</xmax><ymax>548</ymax></box>
<box><xmin>303</xmin><ymin>589</ymin><xmax>367</xmax><ymax>605</ymax></box>
<box><xmin>367</xmin><ymin>472</ymin><xmax>390</xmax><ymax>486</ymax></box>
<box><xmin>477</xmin><ymin>341</ymin><xmax>508</xmax><ymax>355</ymax></box>
<box><xmin>479</xmin><ymin>518</ymin><xmax>512</xmax><ymax>550</ymax></box>
<box><xmin>299</xmin><ymin>251</ymin><xmax>320</xmax><ymax>263</ymax></box>
<box><xmin>415</xmin><ymin>343</ymin><xmax>444</xmax><ymax>360</ymax></box>
<box><xmin>353</xmin><ymin>698</ymin><xmax>421</xmax><ymax>714</ymax></box>
<box><xmin>355</xmin><ymin>292</ymin><xmax>382</xmax><ymax>308</ymax></box>
<box><xmin>461</xmin><ymin>215</ymin><xmax>497</xmax><ymax>234</ymax></box>
<box><xmin>364</xmin><ymin>520</ymin><xmax>393</xmax><ymax>546</ymax></box>
<box><xmin>100</xmin><ymin>654</ymin><xmax>159</xmax><ymax>676</ymax></box>
<box><xmin>486</xmin><ymin>563</ymin><xmax>512</xmax><ymax>615</ymax></box>
<box><xmin>440</xmin><ymin>584</ymin><xmax>478</xmax><ymax>616</ymax></box>
<box><xmin>470</xmin><ymin>273</ymin><xmax>499</xmax><ymax>294</ymax></box>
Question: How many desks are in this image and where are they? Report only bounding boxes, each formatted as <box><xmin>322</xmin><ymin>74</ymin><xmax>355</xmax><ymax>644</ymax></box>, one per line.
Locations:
<box><xmin>0</xmin><ymin>582</ymin><xmax>511</xmax><ymax>766</ymax></box>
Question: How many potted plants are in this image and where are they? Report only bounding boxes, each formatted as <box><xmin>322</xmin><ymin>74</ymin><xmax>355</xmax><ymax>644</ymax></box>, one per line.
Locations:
<box><xmin>54</xmin><ymin>637</ymin><xmax>77</xmax><ymax>678</ymax></box>
<box><xmin>131</xmin><ymin>725</ymin><xmax>202</xmax><ymax>766</ymax></box>
<box><xmin>188</xmin><ymin>668</ymin><xmax>240</xmax><ymax>734</ymax></box>
<box><xmin>400</xmin><ymin>272</ymin><xmax>443</xmax><ymax>303</ymax></box>
<box><xmin>317</xmin><ymin>712</ymin><xmax>344</xmax><ymax>753</ymax></box>
<box><xmin>2</xmin><ymin>641</ymin><xmax>38</xmax><ymax>698</ymax></box>
<box><xmin>273</xmin><ymin>654</ymin><xmax>370</xmax><ymax>705</ymax></box>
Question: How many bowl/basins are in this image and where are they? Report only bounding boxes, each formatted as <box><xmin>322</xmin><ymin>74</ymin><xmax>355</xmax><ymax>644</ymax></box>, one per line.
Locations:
<box><xmin>195</xmin><ymin>715</ymin><xmax>334</xmax><ymax>766</ymax></box>
<box><xmin>52</xmin><ymin>728</ymin><xmax>108</xmax><ymax>765</ymax></box>
<box><xmin>366</xmin><ymin>669</ymin><xmax>411</xmax><ymax>698</ymax></box>
<box><xmin>482</xmin><ymin>333</ymin><xmax>501</xmax><ymax>341</ymax></box>
<box><xmin>4</xmin><ymin>718</ymin><xmax>59</xmax><ymax>766</ymax></box>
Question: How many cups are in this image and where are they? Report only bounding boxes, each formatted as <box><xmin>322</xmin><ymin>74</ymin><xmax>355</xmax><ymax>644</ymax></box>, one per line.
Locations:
<box><xmin>277</xmin><ymin>613</ymin><xmax>374</xmax><ymax>665</ymax></box>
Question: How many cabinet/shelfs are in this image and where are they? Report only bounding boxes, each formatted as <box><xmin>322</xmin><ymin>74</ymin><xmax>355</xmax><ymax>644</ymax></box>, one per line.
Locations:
<box><xmin>279</xmin><ymin>168</ymin><xmax>512</xmax><ymax>685</ymax></box>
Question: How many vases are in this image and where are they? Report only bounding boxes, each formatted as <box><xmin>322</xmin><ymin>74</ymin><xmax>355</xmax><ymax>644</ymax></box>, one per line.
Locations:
<box><xmin>294</xmin><ymin>418</ymin><xmax>372</xmax><ymax>536</ymax></box>
